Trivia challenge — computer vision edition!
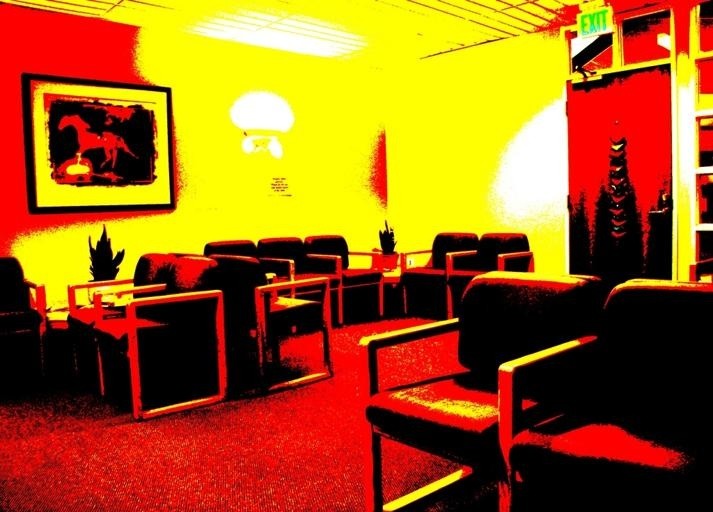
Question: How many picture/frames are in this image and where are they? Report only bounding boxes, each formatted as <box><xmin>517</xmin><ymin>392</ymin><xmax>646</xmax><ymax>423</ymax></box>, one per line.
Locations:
<box><xmin>18</xmin><ymin>71</ymin><xmax>175</xmax><ymax>216</ymax></box>
